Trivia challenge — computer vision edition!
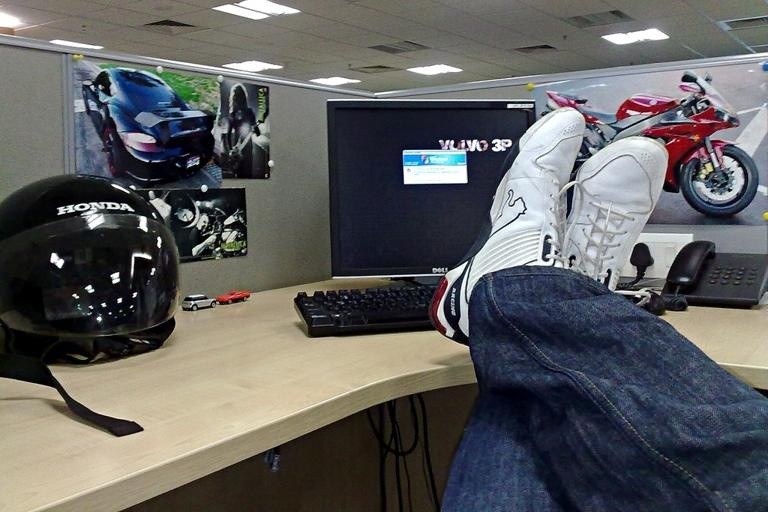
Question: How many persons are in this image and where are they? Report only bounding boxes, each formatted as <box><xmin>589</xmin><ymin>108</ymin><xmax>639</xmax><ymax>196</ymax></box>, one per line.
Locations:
<box><xmin>227</xmin><ymin>83</ymin><xmax>261</xmax><ymax>180</ymax></box>
<box><xmin>431</xmin><ymin>106</ymin><xmax>768</xmax><ymax>512</ymax></box>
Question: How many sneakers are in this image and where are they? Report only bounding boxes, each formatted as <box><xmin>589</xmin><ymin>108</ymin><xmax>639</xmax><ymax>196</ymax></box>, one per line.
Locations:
<box><xmin>566</xmin><ymin>136</ymin><xmax>669</xmax><ymax>307</ymax></box>
<box><xmin>430</xmin><ymin>107</ymin><xmax>585</xmax><ymax>347</ymax></box>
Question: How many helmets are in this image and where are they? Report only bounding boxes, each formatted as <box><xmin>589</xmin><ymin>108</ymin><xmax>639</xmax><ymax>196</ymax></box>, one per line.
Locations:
<box><xmin>0</xmin><ymin>172</ymin><xmax>180</xmax><ymax>364</ymax></box>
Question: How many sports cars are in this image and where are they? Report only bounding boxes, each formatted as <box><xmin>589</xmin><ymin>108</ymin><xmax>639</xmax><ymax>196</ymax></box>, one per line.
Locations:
<box><xmin>82</xmin><ymin>68</ymin><xmax>215</xmax><ymax>186</ymax></box>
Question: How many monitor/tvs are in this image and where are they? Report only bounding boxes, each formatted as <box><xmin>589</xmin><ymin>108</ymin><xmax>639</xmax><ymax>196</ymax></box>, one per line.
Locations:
<box><xmin>325</xmin><ymin>99</ymin><xmax>537</xmax><ymax>287</ymax></box>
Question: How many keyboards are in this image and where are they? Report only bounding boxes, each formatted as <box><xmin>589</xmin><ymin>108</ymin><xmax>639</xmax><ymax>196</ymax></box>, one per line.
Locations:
<box><xmin>293</xmin><ymin>284</ymin><xmax>461</xmax><ymax>339</ymax></box>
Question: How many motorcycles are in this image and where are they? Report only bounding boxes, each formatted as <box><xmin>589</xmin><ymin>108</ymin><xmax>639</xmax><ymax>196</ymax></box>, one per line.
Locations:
<box><xmin>540</xmin><ymin>70</ymin><xmax>758</xmax><ymax>216</ymax></box>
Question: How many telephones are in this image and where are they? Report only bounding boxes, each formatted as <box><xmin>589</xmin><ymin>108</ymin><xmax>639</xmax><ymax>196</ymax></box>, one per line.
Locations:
<box><xmin>660</xmin><ymin>240</ymin><xmax>768</xmax><ymax>311</ymax></box>
